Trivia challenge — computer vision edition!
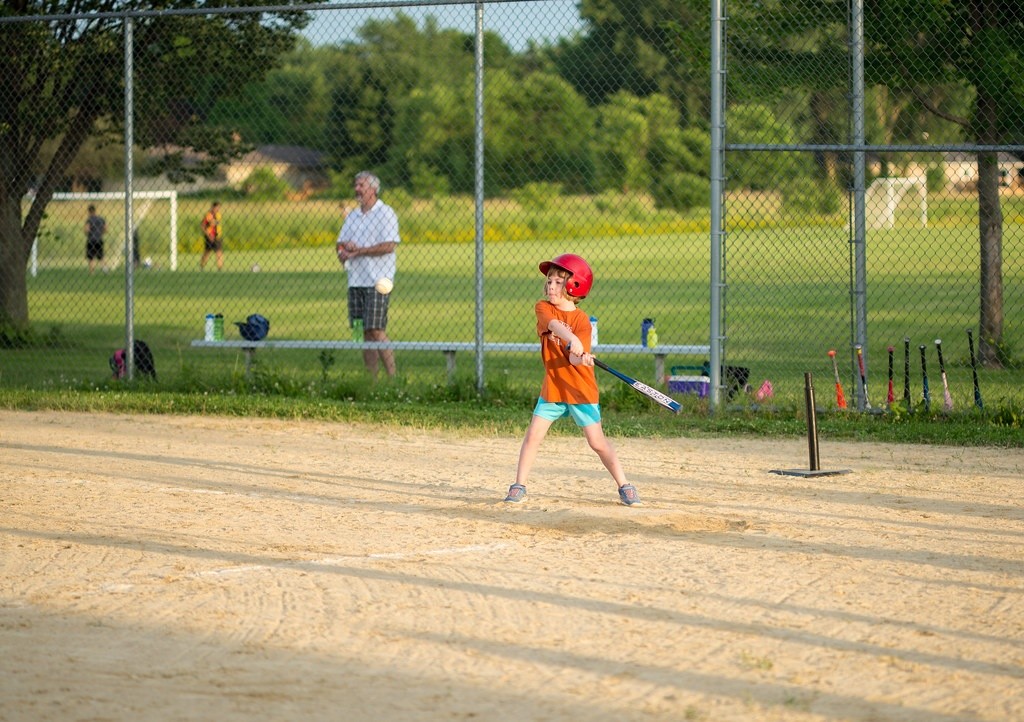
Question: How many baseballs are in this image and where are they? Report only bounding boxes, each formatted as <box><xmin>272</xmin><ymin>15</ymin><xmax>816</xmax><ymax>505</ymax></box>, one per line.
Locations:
<box><xmin>375</xmin><ymin>277</ymin><xmax>395</xmax><ymax>295</ymax></box>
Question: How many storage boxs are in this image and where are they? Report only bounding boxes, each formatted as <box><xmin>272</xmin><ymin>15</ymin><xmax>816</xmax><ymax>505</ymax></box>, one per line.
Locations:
<box><xmin>668</xmin><ymin>375</ymin><xmax>710</xmax><ymax>398</ymax></box>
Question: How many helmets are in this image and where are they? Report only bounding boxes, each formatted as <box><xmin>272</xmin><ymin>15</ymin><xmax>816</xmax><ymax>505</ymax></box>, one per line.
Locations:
<box><xmin>236</xmin><ymin>315</ymin><xmax>269</xmax><ymax>341</ymax></box>
<box><xmin>539</xmin><ymin>254</ymin><xmax>593</xmax><ymax>298</ymax></box>
<box><xmin>110</xmin><ymin>339</ymin><xmax>157</xmax><ymax>383</ymax></box>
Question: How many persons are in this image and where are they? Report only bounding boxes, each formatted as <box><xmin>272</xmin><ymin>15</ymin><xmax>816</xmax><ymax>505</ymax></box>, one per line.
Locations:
<box><xmin>83</xmin><ymin>203</ymin><xmax>108</xmax><ymax>275</ymax></box>
<box><xmin>196</xmin><ymin>200</ymin><xmax>225</xmax><ymax>273</ymax></box>
<box><xmin>335</xmin><ymin>169</ymin><xmax>402</xmax><ymax>386</ymax></box>
<box><xmin>503</xmin><ymin>253</ymin><xmax>643</xmax><ymax>506</ymax></box>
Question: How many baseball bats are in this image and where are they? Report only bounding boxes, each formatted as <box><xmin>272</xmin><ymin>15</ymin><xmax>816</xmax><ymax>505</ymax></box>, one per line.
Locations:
<box><xmin>826</xmin><ymin>351</ymin><xmax>845</xmax><ymax>409</ymax></box>
<box><xmin>903</xmin><ymin>338</ymin><xmax>912</xmax><ymax>405</ymax></box>
<box><xmin>856</xmin><ymin>345</ymin><xmax>871</xmax><ymax>408</ymax></box>
<box><xmin>565</xmin><ymin>341</ymin><xmax>684</xmax><ymax>419</ymax></box>
<box><xmin>966</xmin><ymin>328</ymin><xmax>985</xmax><ymax>409</ymax></box>
<box><xmin>883</xmin><ymin>347</ymin><xmax>893</xmax><ymax>405</ymax></box>
<box><xmin>933</xmin><ymin>339</ymin><xmax>955</xmax><ymax>405</ymax></box>
<box><xmin>919</xmin><ymin>344</ymin><xmax>933</xmax><ymax>404</ymax></box>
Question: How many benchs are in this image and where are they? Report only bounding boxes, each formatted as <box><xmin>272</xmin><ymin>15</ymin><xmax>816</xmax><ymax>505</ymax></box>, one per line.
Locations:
<box><xmin>190</xmin><ymin>339</ymin><xmax>710</xmax><ymax>393</ymax></box>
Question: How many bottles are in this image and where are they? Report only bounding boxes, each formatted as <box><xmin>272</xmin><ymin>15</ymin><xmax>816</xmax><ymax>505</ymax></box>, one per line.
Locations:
<box><xmin>214</xmin><ymin>314</ymin><xmax>224</xmax><ymax>341</ymax></box>
<box><xmin>640</xmin><ymin>317</ymin><xmax>658</xmax><ymax>347</ymax></box>
<box><xmin>204</xmin><ymin>314</ymin><xmax>214</xmax><ymax>341</ymax></box>
<box><xmin>352</xmin><ymin>314</ymin><xmax>364</xmax><ymax>343</ymax></box>
<box><xmin>590</xmin><ymin>316</ymin><xmax>598</xmax><ymax>347</ymax></box>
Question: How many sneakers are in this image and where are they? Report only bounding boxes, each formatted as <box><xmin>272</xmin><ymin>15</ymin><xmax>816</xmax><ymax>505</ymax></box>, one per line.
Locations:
<box><xmin>618</xmin><ymin>483</ymin><xmax>642</xmax><ymax>506</ymax></box>
<box><xmin>504</xmin><ymin>483</ymin><xmax>528</xmax><ymax>504</ymax></box>
